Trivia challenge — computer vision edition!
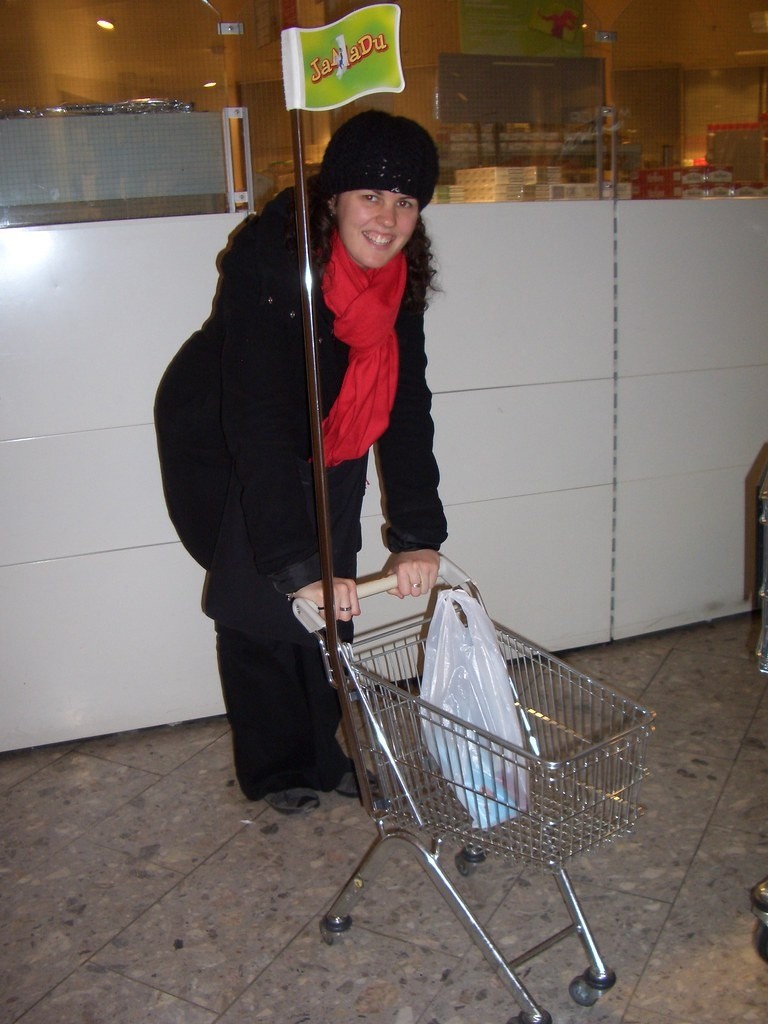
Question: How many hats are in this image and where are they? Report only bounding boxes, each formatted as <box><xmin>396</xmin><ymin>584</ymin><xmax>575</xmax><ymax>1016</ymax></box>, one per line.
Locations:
<box><xmin>317</xmin><ymin>109</ymin><xmax>439</xmax><ymax>215</ymax></box>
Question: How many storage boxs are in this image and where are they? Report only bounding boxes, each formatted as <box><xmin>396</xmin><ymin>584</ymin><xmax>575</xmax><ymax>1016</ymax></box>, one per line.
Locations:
<box><xmin>431</xmin><ymin>166</ymin><xmax>768</xmax><ymax>204</ymax></box>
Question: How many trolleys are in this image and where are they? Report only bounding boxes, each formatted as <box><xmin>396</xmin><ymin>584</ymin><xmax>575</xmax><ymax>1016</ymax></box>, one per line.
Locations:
<box><xmin>293</xmin><ymin>554</ymin><xmax>656</xmax><ymax>1024</ymax></box>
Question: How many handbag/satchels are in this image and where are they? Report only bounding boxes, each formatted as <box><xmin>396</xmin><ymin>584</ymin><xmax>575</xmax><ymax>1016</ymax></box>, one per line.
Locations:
<box><xmin>201</xmin><ymin>449</ymin><xmax>363</xmax><ymax>643</ymax></box>
<box><xmin>419</xmin><ymin>588</ymin><xmax>530</xmax><ymax>829</ymax></box>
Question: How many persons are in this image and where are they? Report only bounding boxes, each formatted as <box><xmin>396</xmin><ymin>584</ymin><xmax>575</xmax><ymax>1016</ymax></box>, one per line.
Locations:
<box><xmin>154</xmin><ymin>109</ymin><xmax>448</xmax><ymax>814</ymax></box>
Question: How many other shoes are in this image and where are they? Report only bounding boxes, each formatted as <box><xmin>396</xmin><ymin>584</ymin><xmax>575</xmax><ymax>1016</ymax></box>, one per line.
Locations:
<box><xmin>265</xmin><ymin>787</ymin><xmax>320</xmax><ymax>817</ymax></box>
<box><xmin>334</xmin><ymin>760</ymin><xmax>380</xmax><ymax>798</ymax></box>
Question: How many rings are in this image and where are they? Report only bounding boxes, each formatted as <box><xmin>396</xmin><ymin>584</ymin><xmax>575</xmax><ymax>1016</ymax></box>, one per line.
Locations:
<box><xmin>317</xmin><ymin>606</ymin><xmax>324</xmax><ymax>610</ymax></box>
<box><xmin>411</xmin><ymin>582</ymin><xmax>421</xmax><ymax>587</ymax></box>
<box><xmin>340</xmin><ymin>606</ymin><xmax>352</xmax><ymax>611</ymax></box>
